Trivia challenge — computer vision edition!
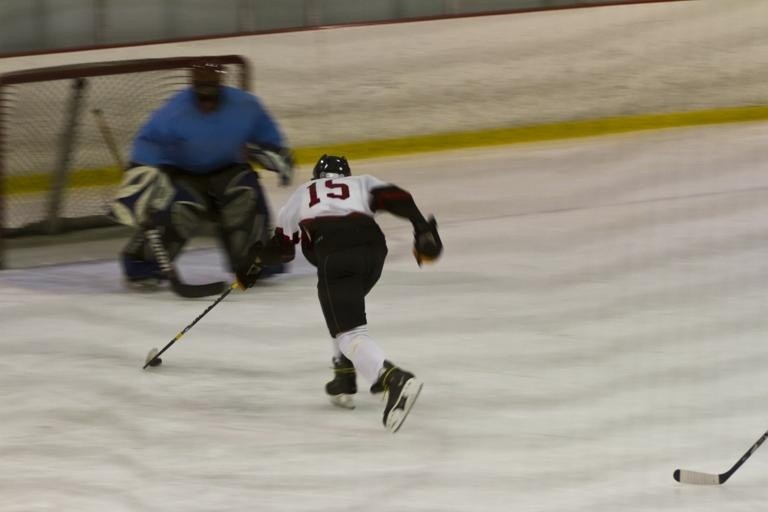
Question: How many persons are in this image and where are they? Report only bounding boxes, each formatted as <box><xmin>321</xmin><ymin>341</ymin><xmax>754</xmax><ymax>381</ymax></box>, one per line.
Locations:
<box><xmin>264</xmin><ymin>152</ymin><xmax>443</xmax><ymax>430</ymax></box>
<box><xmin>103</xmin><ymin>60</ymin><xmax>297</xmax><ymax>283</ymax></box>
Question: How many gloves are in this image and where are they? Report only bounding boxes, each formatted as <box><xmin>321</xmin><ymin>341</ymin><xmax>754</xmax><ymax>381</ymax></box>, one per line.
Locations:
<box><xmin>414</xmin><ymin>216</ymin><xmax>441</xmax><ymax>266</ymax></box>
<box><xmin>235</xmin><ymin>257</ymin><xmax>267</xmax><ymax>293</ymax></box>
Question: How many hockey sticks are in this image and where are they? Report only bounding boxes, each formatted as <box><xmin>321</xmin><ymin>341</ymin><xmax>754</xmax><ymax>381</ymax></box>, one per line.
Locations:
<box><xmin>143</xmin><ymin>282</ymin><xmax>239</xmax><ymax>370</ymax></box>
<box><xmin>674</xmin><ymin>430</ymin><xmax>768</xmax><ymax>484</ymax></box>
<box><xmin>93</xmin><ymin>107</ymin><xmax>226</xmax><ymax>297</ymax></box>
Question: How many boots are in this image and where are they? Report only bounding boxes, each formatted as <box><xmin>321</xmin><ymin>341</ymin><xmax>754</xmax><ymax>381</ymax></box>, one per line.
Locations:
<box><xmin>325</xmin><ymin>356</ymin><xmax>357</xmax><ymax>395</ymax></box>
<box><xmin>370</xmin><ymin>360</ymin><xmax>415</xmax><ymax>426</ymax></box>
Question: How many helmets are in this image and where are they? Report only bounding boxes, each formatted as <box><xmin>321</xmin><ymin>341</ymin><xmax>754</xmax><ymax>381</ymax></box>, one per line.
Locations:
<box><xmin>314</xmin><ymin>153</ymin><xmax>352</xmax><ymax>179</ymax></box>
<box><xmin>191</xmin><ymin>60</ymin><xmax>224</xmax><ymax>111</ymax></box>
